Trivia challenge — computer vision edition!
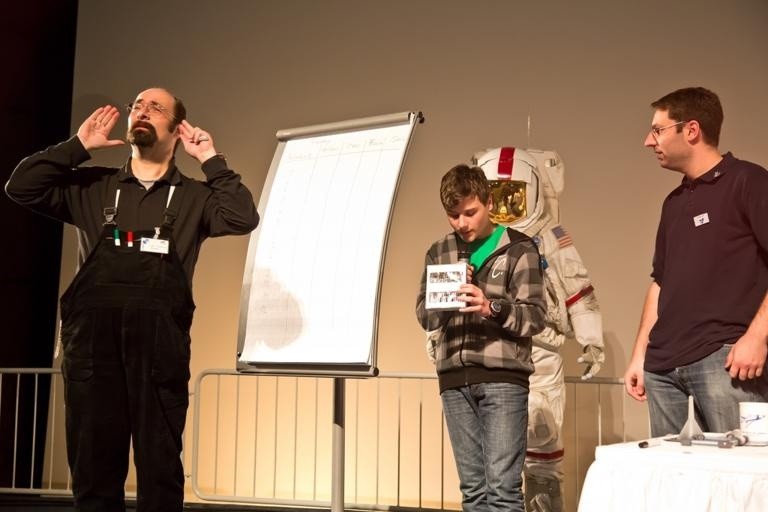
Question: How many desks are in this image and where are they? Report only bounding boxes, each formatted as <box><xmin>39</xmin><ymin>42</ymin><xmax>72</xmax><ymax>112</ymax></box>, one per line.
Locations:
<box><xmin>594</xmin><ymin>432</ymin><xmax>768</xmax><ymax>512</ymax></box>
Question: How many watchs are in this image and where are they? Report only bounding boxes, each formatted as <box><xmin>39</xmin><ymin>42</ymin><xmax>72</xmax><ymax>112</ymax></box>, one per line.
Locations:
<box><xmin>484</xmin><ymin>301</ymin><xmax>502</xmax><ymax>320</ymax></box>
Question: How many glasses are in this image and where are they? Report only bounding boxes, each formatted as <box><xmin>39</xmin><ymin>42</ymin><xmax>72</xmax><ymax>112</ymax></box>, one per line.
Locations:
<box><xmin>126</xmin><ymin>102</ymin><xmax>179</xmax><ymax>123</ymax></box>
<box><xmin>649</xmin><ymin>120</ymin><xmax>689</xmax><ymax>135</ymax></box>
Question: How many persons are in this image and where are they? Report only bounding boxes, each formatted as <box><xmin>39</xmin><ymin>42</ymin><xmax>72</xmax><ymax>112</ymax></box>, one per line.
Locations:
<box><xmin>415</xmin><ymin>161</ymin><xmax>547</xmax><ymax>512</ymax></box>
<box><xmin>4</xmin><ymin>87</ymin><xmax>260</xmax><ymax>511</ymax></box>
<box><xmin>469</xmin><ymin>146</ymin><xmax>605</xmax><ymax>512</ymax></box>
<box><xmin>623</xmin><ymin>85</ymin><xmax>768</xmax><ymax>440</ymax></box>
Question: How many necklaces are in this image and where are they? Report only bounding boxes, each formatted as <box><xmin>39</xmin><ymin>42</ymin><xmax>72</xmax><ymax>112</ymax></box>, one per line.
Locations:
<box><xmin>137</xmin><ymin>178</ymin><xmax>157</xmax><ymax>184</ymax></box>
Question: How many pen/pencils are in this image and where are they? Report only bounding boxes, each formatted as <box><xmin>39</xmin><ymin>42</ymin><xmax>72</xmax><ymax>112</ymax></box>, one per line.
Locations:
<box><xmin>113</xmin><ymin>229</ymin><xmax>119</xmax><ymax>246</ymax></box>
<box><xmin>126</xmin><ymin>231</ymin><xmax>133</xmax><ymax>248</ymax></box>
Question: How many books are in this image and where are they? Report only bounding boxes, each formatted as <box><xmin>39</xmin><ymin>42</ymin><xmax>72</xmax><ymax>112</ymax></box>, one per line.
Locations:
<box><xmin>425</xmin><ymin>263</ymin><xmax>466</xmax><ymax>313</ymax></box>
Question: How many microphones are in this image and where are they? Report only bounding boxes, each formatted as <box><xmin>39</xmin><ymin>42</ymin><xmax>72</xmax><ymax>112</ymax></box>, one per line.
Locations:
<box><xmin>457</xmin><ymin>247</ymin><xmax>471</xmax><ymax>265</ymax></box>
